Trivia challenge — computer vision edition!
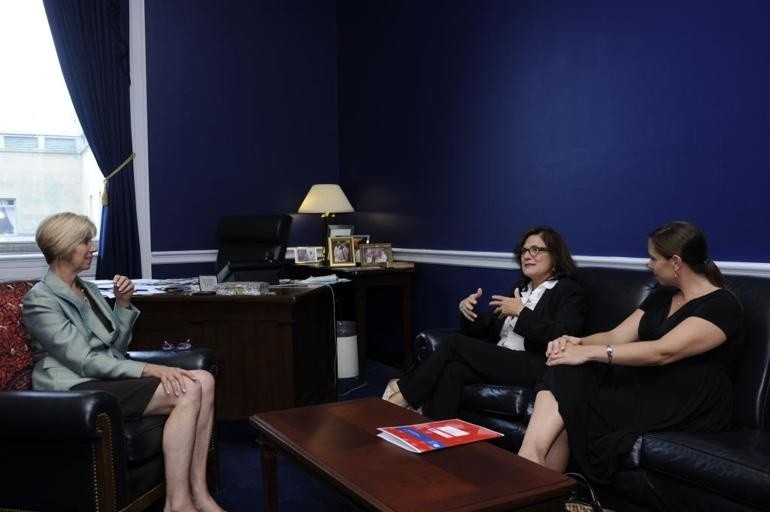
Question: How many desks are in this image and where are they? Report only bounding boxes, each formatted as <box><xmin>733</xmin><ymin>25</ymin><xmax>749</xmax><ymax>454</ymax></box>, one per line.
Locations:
<box><xmin>106</xmin><ymin>279</ymin><xmax>337</xmax><ymax>425</ymax></box>
<box><xmin>320</xmin><ymin>262</ymin><xmax>415</xmax><ymax>385</ymax></box>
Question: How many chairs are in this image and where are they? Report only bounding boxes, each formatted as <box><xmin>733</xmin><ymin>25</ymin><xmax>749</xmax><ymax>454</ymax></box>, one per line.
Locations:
<box><xmin>210</xmin><ymin>214</ymin><xmax>294</xmax><ymax>283</ymax></box>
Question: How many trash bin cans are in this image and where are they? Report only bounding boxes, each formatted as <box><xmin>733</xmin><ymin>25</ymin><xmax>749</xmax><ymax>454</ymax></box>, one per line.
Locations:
<box><xmin>337</xmin><ymin>320</ymin><xmax>359</xmax><ymax>380</ymax></box>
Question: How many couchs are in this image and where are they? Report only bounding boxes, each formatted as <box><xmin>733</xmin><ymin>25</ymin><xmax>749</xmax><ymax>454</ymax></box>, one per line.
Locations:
<box><xmin>1</xmin><ymin>281</ymin><xmax>220</xmax><ymax>512</ymax></box>
<box><xmin>410</xmin><ymin>268</ymin><xmax>770</xmax><ymax>512</ymax></box>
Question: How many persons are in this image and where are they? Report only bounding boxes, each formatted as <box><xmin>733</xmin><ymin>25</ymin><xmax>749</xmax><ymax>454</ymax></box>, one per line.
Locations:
<box><xmin>518</xmin><ymin>220</ymin><xmax>744</xmax><ymax>473</ymax></box>
<box><xmin>387</xmin><ymin>225</ymin><xmax>587</xmax><ymax>419</ymax></box>
<box><xmin>20</xmin><ymin>211</ymin><xmax>225</xmax><ymax>512</ymax></box>
<box><xmin>334</xmin><ymin>241</ymin><xmax>350</xmax><ymax>263</ymax></box>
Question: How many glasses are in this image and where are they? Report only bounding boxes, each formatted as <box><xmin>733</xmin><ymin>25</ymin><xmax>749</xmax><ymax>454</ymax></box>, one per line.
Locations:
<box><xmin>521</xmin><ymin>246</ymin><xmax>549</xmax><ymax>257</ymax></box>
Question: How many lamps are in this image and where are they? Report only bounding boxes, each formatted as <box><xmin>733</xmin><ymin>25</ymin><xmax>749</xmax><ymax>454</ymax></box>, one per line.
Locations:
<box><xmin>298</xmin><ymin>184</ymin><xmax>354</xmax><ymax>266</ymax></box>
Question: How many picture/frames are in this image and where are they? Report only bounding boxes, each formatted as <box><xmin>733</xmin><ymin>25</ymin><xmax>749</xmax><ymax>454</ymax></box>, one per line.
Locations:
<box><xmin>314</xmin><ymin>247</ymin><xmax>326</xmax><ymax>262</ymax></box>
<box><xmin>335</xmin><ymin>235</ymin><xmax>370</xmax><ymax>264</ymax></box>
<box><xmin>360</xmin><ymin>243</ymin><xmax>393</xmax><ymax>266</ymax></box>
<box><xmin>327</xmin><ymin>237</ymin><xmax>356</xmax><ymax>267</ymax></box>
<box><xmin>295</xmin><ymin>247</ymin><xmax>315</xmax><ymax>264</ymax></box>
<box><xmin>327</xmin><ymin>225</ymin><xmax>354</xmax><ymax>237</ymax></box>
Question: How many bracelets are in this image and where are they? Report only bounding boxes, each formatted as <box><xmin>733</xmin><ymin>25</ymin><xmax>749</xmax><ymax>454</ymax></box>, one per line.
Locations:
<box><xmin>606</xmin><ymin>344</ymin><xmax>613</xmax><ymax>364</ymax></box>
